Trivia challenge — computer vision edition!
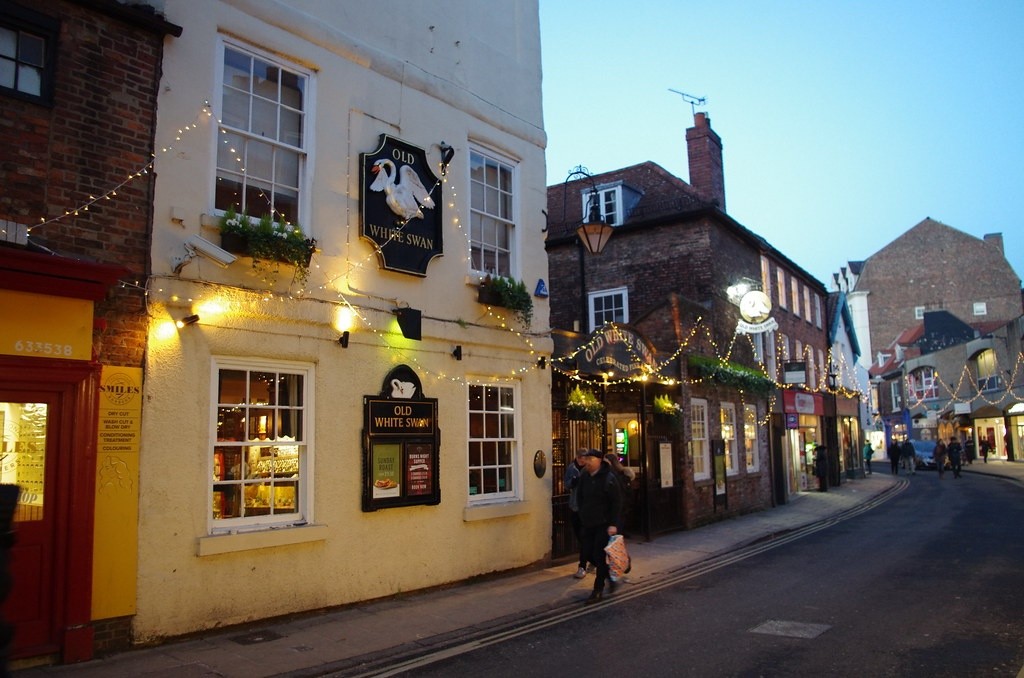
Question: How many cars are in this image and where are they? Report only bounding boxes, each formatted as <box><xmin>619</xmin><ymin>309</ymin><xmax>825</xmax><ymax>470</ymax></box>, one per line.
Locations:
<box><xmin>900</xmin><ymin>438</ymin><xmax>951</xmax><ymax>471</ymax></box>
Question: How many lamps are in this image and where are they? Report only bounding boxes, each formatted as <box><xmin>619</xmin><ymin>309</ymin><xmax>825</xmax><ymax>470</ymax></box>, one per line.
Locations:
<box><xmin>453</xmin><ymin>345</ymin><xmax>462</xmax><ymax>360</ymax></box>
<box><xmin>536</xmin><ymin>355</ymin><xmax>545</xmax><ymax>369</ymax></box>
<box><xmin>339</xmin><ymin>331</ymin><xmax>350</xmax><ymax>348</ymax></box>
<box><xmin>538</xmin><ymin>165</ymin><xmax>614</xmax><ymax>258</ymax></box>
<box><xmin>391</xmin><ymin>300</ymin><xmax>424</xmax><ymax>341</ymax></box>
<box><xmin>178</xmin><ymin>315</ymin><xmax>199</xmax><ymax>328</ymax></box>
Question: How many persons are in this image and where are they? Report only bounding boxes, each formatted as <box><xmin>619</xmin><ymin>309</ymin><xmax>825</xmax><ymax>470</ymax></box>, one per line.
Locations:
<box><xmin>900</xmin><ymin>439</ymin><xmax>916</xmax><ymax>475</ymax></box>
<box><xmin>947</xmin><ymin>436</ymin><xmax>963</xmax><ymax>478</ymax></box>
<box><xmin>863</xmin><ymin>440</ymin><xmax>874</xmax><ymax>475</ymax></box>
<box><xmin>980</xmin><ymin>435</ymin><xmax>993</xmax><ymax>463</ymax></box>
<box><xmin>576</xmin><ymin>449</ymin><xmax>621</xmax><ymax>604</ymax></box>
<box><xmin>964</xmin><ymin>436</ymin><xmax>975</xmax><ymax>463</ymax></box>
<box><xmin>565</xmin><ymin>450</ymin><xmax>595</xmax><ymax>579</ymax></box>
<box><xmin>887</xmin><ymin>438</ymin><xmax>901</xmax><ymax>475</ymax></box>
<box><xmin>603</xmin><ymin>453</ymin><xmax>635</xmax><ymax>582</ymax></box>
<box><xmin>813</xmin><ymin>445</ymin><xmax>828</xmax><ymax>493</ymax></box>
<box><xmin>933</xmin><ymin>439</ymin><xmax>947</xmax><ymax>479</ymax></box>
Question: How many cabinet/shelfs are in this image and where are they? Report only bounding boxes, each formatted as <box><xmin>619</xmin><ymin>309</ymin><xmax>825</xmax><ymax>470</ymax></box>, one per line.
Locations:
<box><xmin>245</xmin><ymin>470</ymin><xmax>298</xmax><ymax>516</ymax></box>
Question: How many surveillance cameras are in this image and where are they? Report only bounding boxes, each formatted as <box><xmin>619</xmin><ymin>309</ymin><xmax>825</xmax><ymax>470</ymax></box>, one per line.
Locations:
<box><xmin>184</xmin><ymin>234</ymin><xmax>237</xmax><ymax>269</ymax></box>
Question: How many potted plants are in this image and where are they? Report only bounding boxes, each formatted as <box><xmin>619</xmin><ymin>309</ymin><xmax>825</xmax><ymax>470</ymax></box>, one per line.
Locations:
<box><xmin>477</xmin><ymin>273</ymin><xmax>534</xmax><ymax>334</ymax></box>
<box><xmin>219</xmin><ymin>204</ymin><xmax>315</xmax><ymax>293</ymax></box>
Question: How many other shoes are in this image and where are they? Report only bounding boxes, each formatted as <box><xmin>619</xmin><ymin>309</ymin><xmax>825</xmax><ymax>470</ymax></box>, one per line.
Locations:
<box><xmin>575</xmin><ymin>566</ymin><xmax>585</xmax><ymax>579</ymax></box>
<box><xmin>866</xmin><ymin>473</ymin><xmax>872</xmax><ymax>475</ymax></box>
<box><xmin>958</xmin><ymin>473</ymin><xmax>961</xmax><ymax>478</ymax></box>
<box><xmin>588</xmin><ymin>563</ymin><xmax>596</xmax><ymax>574</ymax></box>
<box><xmin>955</xmin><ymin>475</ymin><xmax>957</xmax><ymax>479</ymax></box>
<box><xmin>587</xmin><ymin>591</ymin><xmax>602</xmax><ymax>602</ymax></box>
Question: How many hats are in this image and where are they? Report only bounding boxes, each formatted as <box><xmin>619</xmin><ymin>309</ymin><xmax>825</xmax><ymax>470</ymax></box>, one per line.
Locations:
<box><xmin>582</xmin><ymin>449</ymin><xmax>603</xmax><ymax>460</ymax></box>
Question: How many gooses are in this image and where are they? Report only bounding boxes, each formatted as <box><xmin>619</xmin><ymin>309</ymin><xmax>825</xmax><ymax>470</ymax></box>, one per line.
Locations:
<box><xmin>370</xmin><ymin>158</ymin><xmax>434</xmax><ymax>225</ymax></box>
<box><xmin>391</xmin><ymin>379</ymin><xmax>416</xmax><ymax>398</ymax></box>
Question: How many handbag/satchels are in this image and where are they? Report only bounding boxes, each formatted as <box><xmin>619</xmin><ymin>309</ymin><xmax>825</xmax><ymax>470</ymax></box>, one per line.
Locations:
<box><xmin>867</xmin><ymin>449</ymin><xmax>874</xmax><ymax>455</ymax></box>
<box><xmin>605</xmin><ymin>533</ymin><xmax>632</xmax><ymax>582</ymax></box>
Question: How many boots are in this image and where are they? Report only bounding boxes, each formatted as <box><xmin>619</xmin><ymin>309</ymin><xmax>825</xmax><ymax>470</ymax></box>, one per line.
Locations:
<box><xmin>940</xmin><ymin>472</ymin><xmax>944</xmax><ymax>480</ymax></box>
